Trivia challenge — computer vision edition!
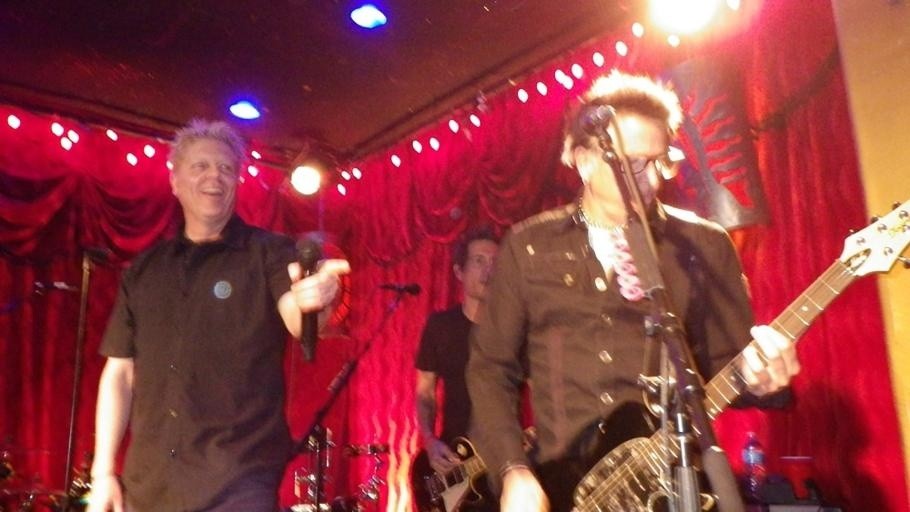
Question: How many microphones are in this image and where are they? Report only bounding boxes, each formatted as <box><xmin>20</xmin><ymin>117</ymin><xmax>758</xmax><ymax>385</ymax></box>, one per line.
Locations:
<box><xmin>295</xmin><ymin>233</ymin><xmax>324</xmax><ymax>362</ymax></box>
<box><xmin>379</xmin><ymin>282</ymin><xmax>422</xmax><ymax>296</ymax></box>
<box><xmin>579</xmin><ymin>104</ymin><xmax>615</xmax><ymax>137</ymax></box>
<box><xmin>82</xmin><ymin>244</ymin><xmax>121</xmax><ymax>269</ymax></box>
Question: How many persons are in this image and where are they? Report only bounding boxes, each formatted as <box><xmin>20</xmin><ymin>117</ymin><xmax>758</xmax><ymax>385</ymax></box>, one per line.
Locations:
<box><xmin>85</xmin><ymin>121</ymin><xmax>351</xmax><ymax>512</ymax></box>
<box><xmin>463</xmin><ymin>73</ymin><xmax>800</xmax><ymax>512</ymax></box>
<box><xmin>415</xmin><ymin>228</ymin><xmax>529</xmax><ymax>476</ymax></box>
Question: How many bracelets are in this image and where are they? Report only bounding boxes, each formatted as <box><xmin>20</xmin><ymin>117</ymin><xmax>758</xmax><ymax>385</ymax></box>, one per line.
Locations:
<box><xmin>497</xmin><ymin>461</ymin><xmax>529</xmax><ymax>477</ymax></box>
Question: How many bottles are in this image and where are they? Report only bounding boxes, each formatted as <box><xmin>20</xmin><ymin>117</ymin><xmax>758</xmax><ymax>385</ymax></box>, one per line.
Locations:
<box><xmin>741</xmin><ymin>430</ymin><xmax>768</xmax><ymax>502</ymax></box>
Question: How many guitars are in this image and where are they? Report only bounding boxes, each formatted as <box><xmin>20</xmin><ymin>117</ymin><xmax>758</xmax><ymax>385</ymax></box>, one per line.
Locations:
<box><xmin>484</xmin><ymin>195</ymin><xmax>910</xmax><ymax>512</ymax></box>
<box><xmin>408</xmin><ymin>421</ymin><xmax>540</xmax><ymax>512</ymax></box>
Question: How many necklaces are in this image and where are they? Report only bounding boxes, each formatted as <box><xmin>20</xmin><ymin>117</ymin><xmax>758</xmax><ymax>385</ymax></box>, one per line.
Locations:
<box><xmin>577</xmin><ymin>194</ymin><xmax>630</xmax><ymax>232</ymax></box>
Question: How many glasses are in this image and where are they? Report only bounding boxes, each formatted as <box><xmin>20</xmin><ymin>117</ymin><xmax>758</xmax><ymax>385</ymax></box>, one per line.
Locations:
<box><xmin>619</xmin><ymin>153</ymin><xmax>680</xmax><ymax>180</ymax></box>
<box><xmin>471</xmin><ymin>254</ymin><xmax>496</xmax><ymax>268</ymax></box>
